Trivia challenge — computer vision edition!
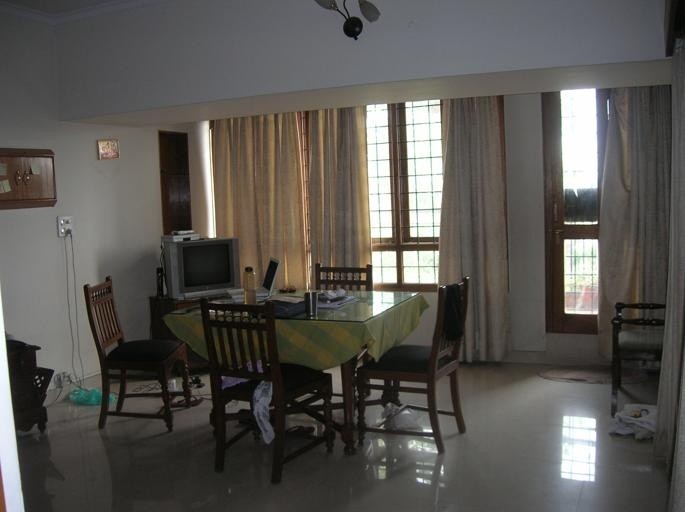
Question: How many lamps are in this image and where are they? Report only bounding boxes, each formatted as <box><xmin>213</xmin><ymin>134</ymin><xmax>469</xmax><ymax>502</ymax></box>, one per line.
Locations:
<box><xmin>315</xmin><ymin>0</ymin><xmax>380</xmax><ymax>40</ymax></box>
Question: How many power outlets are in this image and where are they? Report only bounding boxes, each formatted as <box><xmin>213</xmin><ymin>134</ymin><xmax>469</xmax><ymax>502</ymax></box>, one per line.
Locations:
<box><xmin>55</xmin><ymin>371</ymin><xmax>73</xmax><ymax>386</ymax></box>
<box><xmin>57</xmin><ymin>213</ymin><xmax>74</xmax><ymax>239</ymax></box>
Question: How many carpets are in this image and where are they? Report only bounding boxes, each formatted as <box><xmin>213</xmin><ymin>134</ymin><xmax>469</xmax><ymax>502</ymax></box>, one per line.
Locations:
<box><xmin>536</xmin><ymin>363</ymin><xmax>648</xmax><ymax>386</ymax></box>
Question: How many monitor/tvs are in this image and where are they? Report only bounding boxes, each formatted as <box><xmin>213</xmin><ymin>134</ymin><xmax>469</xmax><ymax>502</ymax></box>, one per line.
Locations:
<box><xmin>162</xmin><ymin>238</ymin><xmax>241</xmax><ymax>301</ymax></box>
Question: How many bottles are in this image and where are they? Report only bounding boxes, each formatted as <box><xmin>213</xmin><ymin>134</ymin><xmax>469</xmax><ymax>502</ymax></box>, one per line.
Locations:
<box><xmin>243</xmin><ymin>266</ymin><xmax>257</xmax><ymax>305</ymax></box>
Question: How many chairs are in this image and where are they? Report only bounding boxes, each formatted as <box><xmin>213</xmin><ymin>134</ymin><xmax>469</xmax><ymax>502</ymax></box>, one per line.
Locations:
<box><xmin>610</xmin><ymin>302</ymin><xmax>666</xmax><ymax>418</ymax></box>
<box><xmin>357</xmin><ymin>275</ymin><xmax>470</xmax><ymax>454</ymax></box>
<box><xmin>199</xmin><ymin>296</ymin><xmax>336</xmax><ymax>485</ymax></box>
<box><xmin>5</xmin><ymin>339</ymin><xmax>54</xmax><ymax>434</ymax></box>
<box><xmin>315</xmin><ymin>263</ymin><xmax>374</xmax><ymax>292</ymax></box>
<box><xmin>83</xmin><ymin>275</ymin><xmax>193</xmax><ymax>433</ymax></box>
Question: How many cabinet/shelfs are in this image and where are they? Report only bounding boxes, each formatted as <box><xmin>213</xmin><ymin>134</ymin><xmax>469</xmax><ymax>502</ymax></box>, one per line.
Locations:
<box><xmin>0</xmin><ymin>149</ymin><xmax>58</xmax><ymax>210</ymax></box>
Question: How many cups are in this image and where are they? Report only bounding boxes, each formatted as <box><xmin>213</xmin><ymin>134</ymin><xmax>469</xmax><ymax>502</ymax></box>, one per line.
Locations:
<box><xmin>304</xmin><ymin>292</ymin><xmax>318</xmax><ymax>317</ymax></box>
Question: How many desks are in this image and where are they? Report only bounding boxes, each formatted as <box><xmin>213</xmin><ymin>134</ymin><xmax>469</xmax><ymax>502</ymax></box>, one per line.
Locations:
<box><xmin>163</xmin><ymin>291</ymin><xmax>423</xmax><ymax>455</ymax></box>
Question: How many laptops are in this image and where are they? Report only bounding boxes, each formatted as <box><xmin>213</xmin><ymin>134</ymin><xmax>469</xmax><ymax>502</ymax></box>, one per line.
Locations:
<box><xmin>227</xmin><ymin>256</ymin><xmax>280</xmax><ymax>301</ymax></box>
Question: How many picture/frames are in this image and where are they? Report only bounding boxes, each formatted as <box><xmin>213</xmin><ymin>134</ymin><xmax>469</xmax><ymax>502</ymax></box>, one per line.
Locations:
<box><xmin>97</xmin><ymin>138</ymin><xmax>120</xmax><ymax>160</ymax></box>
